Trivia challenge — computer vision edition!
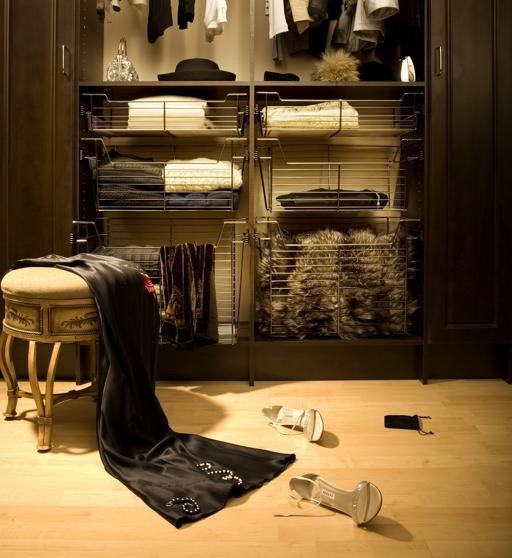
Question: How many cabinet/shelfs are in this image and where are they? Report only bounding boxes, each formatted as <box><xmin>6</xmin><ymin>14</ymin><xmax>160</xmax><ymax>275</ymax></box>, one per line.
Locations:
<box><xmin>73</xmin><ymin>0</ymin><xmax>427</xmax><ymax>385</ymax></box>
<box><xmin>0</xmin><ymin>2</ymin><xmax>103</xmax><ymax>382</ymax></box>
<box><xmin>428</xmin><ymin>0</ymin><xmax>512</xmax><ymax>383</ymax></box>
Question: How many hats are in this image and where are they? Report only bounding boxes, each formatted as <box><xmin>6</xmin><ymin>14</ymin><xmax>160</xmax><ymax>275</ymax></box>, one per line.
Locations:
<box><xmin>157</xmin><ymin>58</ymin><xmax>236</xmax><ymax>82</ymax></box>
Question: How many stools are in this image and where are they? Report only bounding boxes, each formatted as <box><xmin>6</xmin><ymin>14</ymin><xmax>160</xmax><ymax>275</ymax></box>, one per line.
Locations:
<box><xmin>1</xmin><ymin>266</ymin><xmax>102</xmax><ymax>453</ymax></box>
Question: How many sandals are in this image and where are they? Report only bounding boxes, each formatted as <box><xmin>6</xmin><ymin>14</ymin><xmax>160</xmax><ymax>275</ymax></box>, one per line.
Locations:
<box><xmin>273</xmin><ymin>472</ymin><xmax>382</xmax><ymax>526</ymax></box>
<box><xmin>260</xmin><ymin>404</ymin><xmax>324</xmax><ymax>443</ymax></box>
